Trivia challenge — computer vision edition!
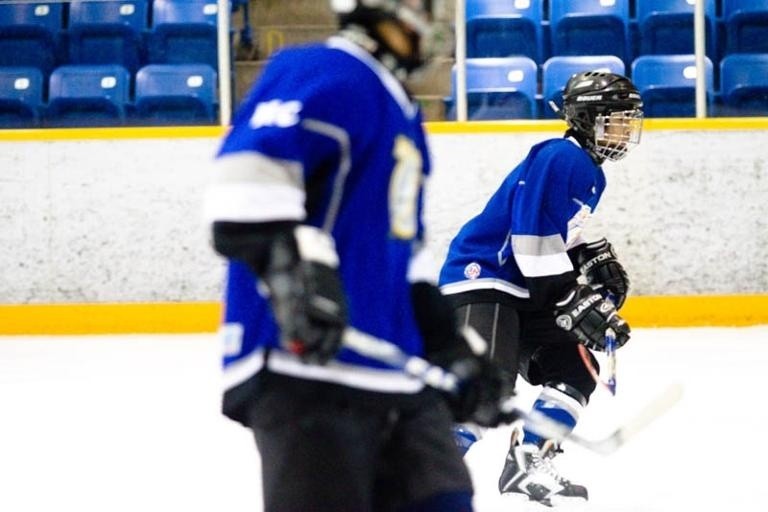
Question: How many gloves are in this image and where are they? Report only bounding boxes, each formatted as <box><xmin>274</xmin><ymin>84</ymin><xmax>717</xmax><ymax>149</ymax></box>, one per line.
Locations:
<box><xmin>551</xmin><ymin>284</ymin><xmax>631</xmax><ymax>353</ymax></box>
<box><xmin>251</xmin><ymin>219</ymin><xmax>348</xmax><ymax>364</ymax></box>
<box><xmin>573</xmin><ymin>237</ymin><xmax>632</xmax><ymax>310</ymax></box>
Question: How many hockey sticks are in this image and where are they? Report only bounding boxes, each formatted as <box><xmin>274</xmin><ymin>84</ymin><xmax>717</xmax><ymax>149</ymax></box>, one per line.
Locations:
<box><xmin>340</xmin><ymin>328</ymin><xmax>684</xmax><ymax>454</ymax></box>
<box><xmin>579</xmin><ymin>328</ymin><xmax>616</xmax><ymax>399</ymax></box>
<box><xmin>343</xmin><ymin>329</ymin><xmax>684</xmax><ymax>453</ymax></box>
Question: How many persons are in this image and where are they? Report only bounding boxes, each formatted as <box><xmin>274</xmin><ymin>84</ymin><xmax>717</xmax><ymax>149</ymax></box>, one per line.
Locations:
<box><xmin>203</xmin><ymin>0</ymin><xmax>474</xmax><ymax>512</ymax></box>
<box><xmin>436</xmin><ymin>70</ymin><xmax>645</xmax><ymax>507</ymax></box>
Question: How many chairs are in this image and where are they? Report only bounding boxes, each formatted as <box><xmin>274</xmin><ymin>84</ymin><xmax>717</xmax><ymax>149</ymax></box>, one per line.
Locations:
<box><xmin>0</xmin><ymin>0</ymin><xmax>236</xmax><ymax>129</ymax></box>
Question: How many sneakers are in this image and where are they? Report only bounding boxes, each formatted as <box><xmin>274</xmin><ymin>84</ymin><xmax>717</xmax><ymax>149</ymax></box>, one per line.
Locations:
<box><xmin>496</xmin><ymin>443</ymin><xmax>589</xmax><ymax>502</ymax></box>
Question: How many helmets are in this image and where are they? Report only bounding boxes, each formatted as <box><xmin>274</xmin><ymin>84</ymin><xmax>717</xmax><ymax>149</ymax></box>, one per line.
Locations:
<box><xmin>336</xmin><ymin>0</ymin><xmax>440</xmax><ymax>81</ymax></box>
<box><xmin>560</xmin><ymin>70</ymin><xmax>644</xmax><ymax>139</ymax></box>
